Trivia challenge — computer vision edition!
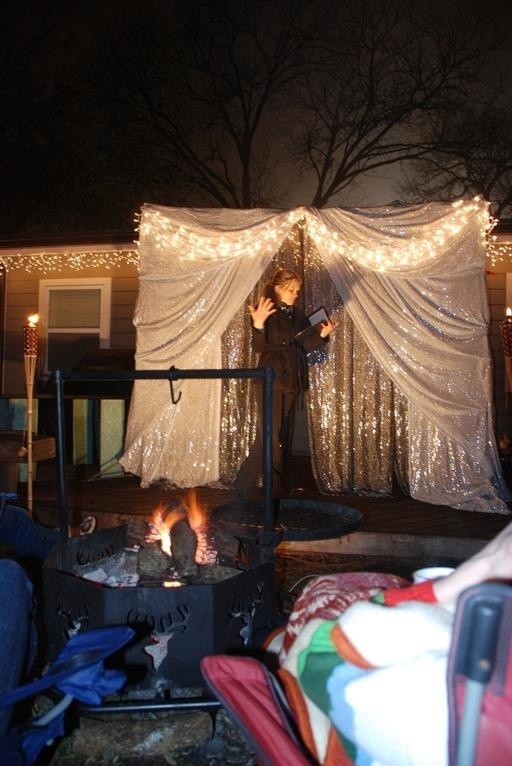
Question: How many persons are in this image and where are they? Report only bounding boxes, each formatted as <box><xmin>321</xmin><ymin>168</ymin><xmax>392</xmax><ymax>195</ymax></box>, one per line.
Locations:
<box><xmin>236</xmin><ymin>265</ymin><xmax>340</xmax><ymax>496</ymax></box>
